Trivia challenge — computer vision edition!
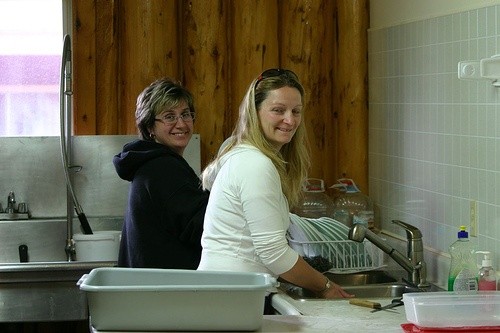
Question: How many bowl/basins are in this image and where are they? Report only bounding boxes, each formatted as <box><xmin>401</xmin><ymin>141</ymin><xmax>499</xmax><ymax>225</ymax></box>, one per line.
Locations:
<box><xmin>402</xmin><ymin>290</ymin><xmax>500</xmax><ymax>330</ymax></box>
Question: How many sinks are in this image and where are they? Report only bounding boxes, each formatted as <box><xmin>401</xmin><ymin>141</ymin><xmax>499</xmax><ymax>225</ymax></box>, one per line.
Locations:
<box><xmin>283</xmin><ymin>285</ymin><xmax>428</xmax><ymax>299</ymax></box>
<box><xmin>0</xmin><ymin>255</ymin><xmax>73</xmax><ymax>265</ymax></box>
<box><xmin>269</xmin><ymin>270</ymin><xmax>397</xmax><ymax>284</ymax></box>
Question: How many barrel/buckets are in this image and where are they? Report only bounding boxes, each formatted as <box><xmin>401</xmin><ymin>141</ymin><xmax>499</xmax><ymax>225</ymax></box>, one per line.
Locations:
<box><xmin>73</xmin><ymin>230</ymin><xmax>121</xmax><ymax>262</ymax></box>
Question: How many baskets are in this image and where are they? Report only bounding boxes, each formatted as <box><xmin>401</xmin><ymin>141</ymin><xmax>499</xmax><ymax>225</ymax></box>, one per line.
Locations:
<box><xmin>286</xmin><ymin>230</ymin><xmax>386</xmax><ymax>274</ymax></box>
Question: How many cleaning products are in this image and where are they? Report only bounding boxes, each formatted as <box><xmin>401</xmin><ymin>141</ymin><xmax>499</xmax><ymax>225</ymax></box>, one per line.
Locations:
<box><xmin>447</xmin><ymin>225</ymin><xmax>478</xmax><ymax>290</ymax></box>
<box><xmin>476</xmin><ymin>251</ymin><xmax>499</xmax><ymax>291</ymax></box>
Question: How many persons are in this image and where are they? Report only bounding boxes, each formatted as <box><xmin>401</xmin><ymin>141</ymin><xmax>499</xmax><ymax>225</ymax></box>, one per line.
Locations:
<box><xmin>112</xmin><ymin>80</ymin><xmax>210</xmax><ymax>273</ymax></box>
<box><xmin>196</xmin><ymin>69</ymin><xmax>356</xmax><ymax>317</ymax></box>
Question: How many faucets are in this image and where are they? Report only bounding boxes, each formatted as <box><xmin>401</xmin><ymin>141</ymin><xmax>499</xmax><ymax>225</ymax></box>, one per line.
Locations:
<box><xmin>349</xmin><ymin>218</ymin><xmax>429</xmax><ymax>286</ymax></box>
<box><xmin>6</xmin><ymin>191</ymin><xmax>17</xmax><ymax>215</ymax></box>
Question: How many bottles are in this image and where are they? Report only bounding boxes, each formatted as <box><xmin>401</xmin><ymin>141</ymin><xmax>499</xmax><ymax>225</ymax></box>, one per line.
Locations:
<box><xmin>294</xmin><ymin>177</ymin><xmax>333</xmax><ymax>219</ymax></box>
<box><xmin>332</xmin><ymin>178</ymin><xmax>375</xmax><ymax>233</ymax></box>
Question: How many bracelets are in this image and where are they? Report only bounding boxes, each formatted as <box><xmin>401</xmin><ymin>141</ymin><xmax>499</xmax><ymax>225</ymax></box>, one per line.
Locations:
<box><xmin>315</xmin><ymin>277</ymin><xmax>330</xmax><ymax>294</ymax></box>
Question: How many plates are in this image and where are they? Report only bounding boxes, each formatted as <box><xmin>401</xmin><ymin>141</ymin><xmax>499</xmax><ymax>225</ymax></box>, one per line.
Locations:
<box><xmin>289</xmin><ymin>210</ymin><xmax>372</xmax><ymax>268</ymax></box>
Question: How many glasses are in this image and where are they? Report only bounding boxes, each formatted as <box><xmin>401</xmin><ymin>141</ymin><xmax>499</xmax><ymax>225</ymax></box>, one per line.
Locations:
<box><xmin>258</xmin><ymin>68</ymin><xmax>299</xmax><ymax>81</ymax></box>
<box><xmin>156</xmin><ymin>112</ymin><xmax>196</xmax><ymax>124</ymax></box>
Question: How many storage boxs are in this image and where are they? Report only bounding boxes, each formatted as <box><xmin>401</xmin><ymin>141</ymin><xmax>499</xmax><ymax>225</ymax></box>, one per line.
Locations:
<box><xmin>401</xmin><ymin>291</ymin><xmax>500</xmax><ymax>328</ymax></box>
